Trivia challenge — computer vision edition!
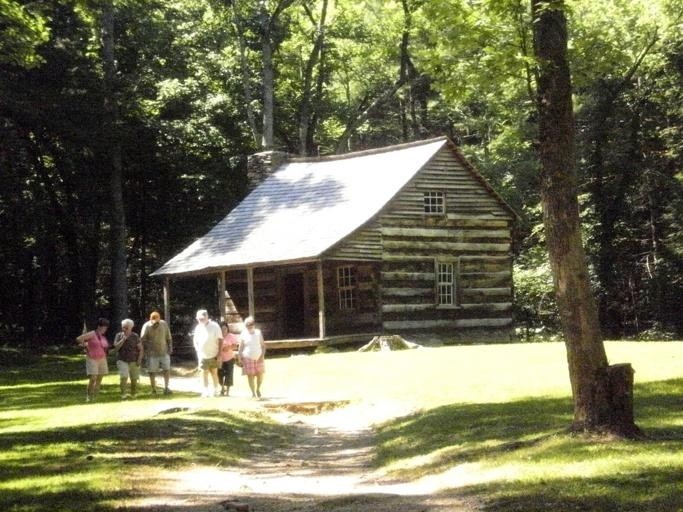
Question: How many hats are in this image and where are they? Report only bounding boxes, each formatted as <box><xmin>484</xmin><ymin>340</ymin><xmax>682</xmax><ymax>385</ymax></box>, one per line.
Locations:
<box><xmin>150</xmin><ymin>312</ymin><xmax>160</xmax><ymax>324</ymax></box>
<box><xmin>196</xmin><ymin>310</ymin><xmax>208</xmax><ymax>319</ymax></box>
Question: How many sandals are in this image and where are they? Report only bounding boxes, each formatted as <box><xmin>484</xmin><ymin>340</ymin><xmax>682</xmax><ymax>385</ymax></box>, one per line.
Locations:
<box><xmin>252</xmin><ymin>391</ymin><xmax>262</xmax><ymax>397</ymax></box>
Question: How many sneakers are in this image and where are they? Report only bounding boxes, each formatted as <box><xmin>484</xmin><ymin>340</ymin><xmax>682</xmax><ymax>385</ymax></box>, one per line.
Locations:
<box><xmin>164</xmin><ymin>387</ymin><xmax>172</xmax><ymax>394</ymax></box>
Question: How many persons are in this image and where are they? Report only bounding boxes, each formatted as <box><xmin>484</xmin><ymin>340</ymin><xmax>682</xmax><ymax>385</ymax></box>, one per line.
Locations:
<box><xmin>75</xmin><ymin>311</ymin><xmax>173</xmax><ymax>402</ymax></box>
<box><xmin>193</xmin><ymin>309</ymin><xmax>266</xmax><ymax>399</ymax></box>
